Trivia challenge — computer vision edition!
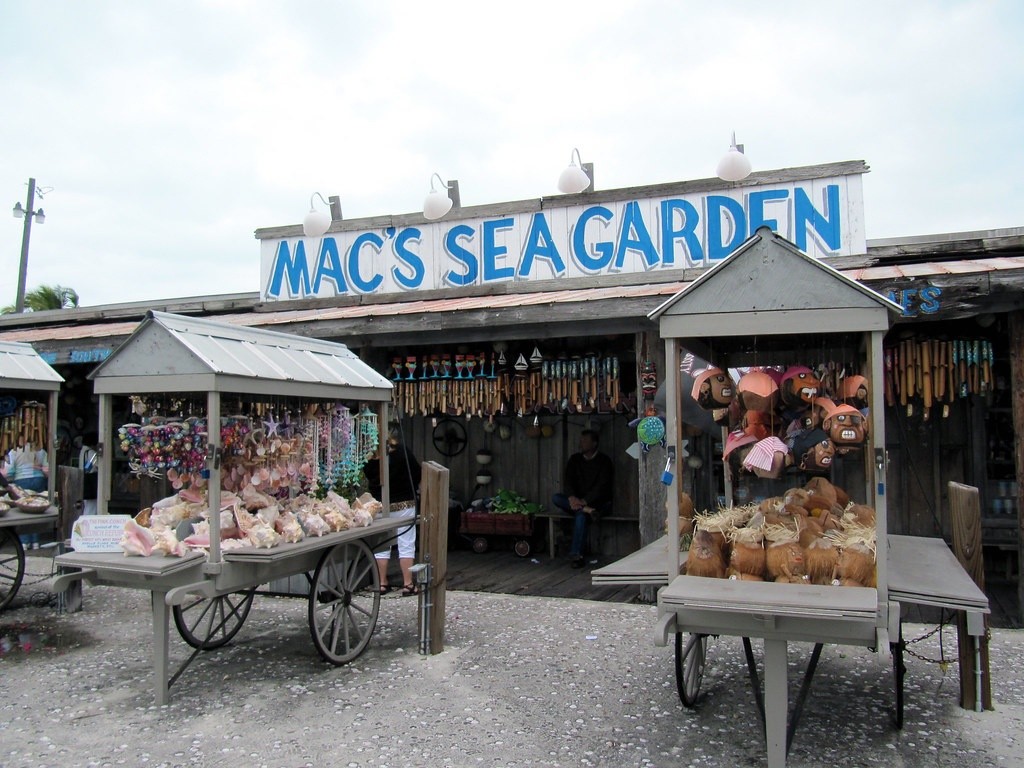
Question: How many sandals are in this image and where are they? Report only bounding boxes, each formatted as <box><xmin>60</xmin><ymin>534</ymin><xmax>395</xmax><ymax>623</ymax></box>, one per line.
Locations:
<box><xmin>402</xmin><ymin>582</ymin><xmax>419</xmax><ymax>596</ymax></box>
<box><xmin>380</xmin><ymin>584</ymin><xmax>392</xmax><ymax>595</ymax></box>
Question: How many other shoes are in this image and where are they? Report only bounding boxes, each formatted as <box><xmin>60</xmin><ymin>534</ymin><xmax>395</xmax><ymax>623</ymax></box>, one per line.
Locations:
<box><xmin>23</xmin><ymin>543</ymin><xmax>32</xmax><ymax>550</ymax></box>
<box><xmin>32</xmin><ymin>543</ymin><xmax>40</xmax><ymax>550</ymax></box>
<box><xmin>571</xmin><ymin>558</ymin><xmax>587</xmax><ymax>568</ymax></box>
<box><xmin>590</xmin><ymin>508</ymin><xmax>599</xmax><ymax>522</ymax></box>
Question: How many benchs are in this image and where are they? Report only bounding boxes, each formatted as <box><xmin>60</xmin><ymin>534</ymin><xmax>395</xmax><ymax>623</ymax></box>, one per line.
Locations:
<box><xmin>534</xmin><ymin>507</ymin><xmax>639</xmax><ymax>561</ymax></box>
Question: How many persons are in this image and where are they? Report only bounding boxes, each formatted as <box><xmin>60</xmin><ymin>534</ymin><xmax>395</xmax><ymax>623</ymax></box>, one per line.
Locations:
<box><xmin>78</xmin><ymin>433</ymin><xmax>99</xmax><ymax>515</ymax></box>
<box><xmin>552</xmin><ymin>429</ymin><xmax>614</xmax><ymax>568</ymax></box>
<box><xmin>363</xmin><ymin>422</ymin><xmax>422</xmax><ymax>597</ymax></box>
<box><xmin>4</xmin><ymin>432</ymin><xmax>49</xmax><ymax>549</ymax></box>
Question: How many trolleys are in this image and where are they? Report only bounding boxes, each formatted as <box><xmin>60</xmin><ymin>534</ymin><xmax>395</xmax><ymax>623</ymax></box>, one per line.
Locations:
<box><xmin>170</xmin><ymin>509</ymin><xmax>431</xmax><ymax>664</ymax></box>
<box><xmin>0</xmin><ymin>490</ymin><xmax>70</xmax><ymax>612</ymax></box>
<box><xmin>655</xmin><ymin>536</ymin><xmax>908</xmax><ymax>731</ymax></box>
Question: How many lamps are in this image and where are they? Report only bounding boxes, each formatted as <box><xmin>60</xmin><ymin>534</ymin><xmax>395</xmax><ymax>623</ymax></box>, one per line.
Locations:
<box><xmin>421</xmin><ymin>173</ymin><xmax>454</xmax><ymax>221</ymax></box>
<box><xmin>714</xmin><ymin>131</ymin><xmax>752</xmax><ymax>181</ymax></box>
<box><xmin>302</xmin><ymin>191</ymin><xmax>336</xmax><ymax>240</ymax></box>
<box><xmin>557</xmin><ymin>147</ymin><xmax>591</xmax><ymax>196</ymax></box>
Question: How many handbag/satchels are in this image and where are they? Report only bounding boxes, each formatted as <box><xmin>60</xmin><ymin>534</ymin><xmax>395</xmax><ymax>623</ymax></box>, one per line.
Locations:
<box><xmin>84</xmin><ymin>471</ymin><xmax>98</xmax><ymax>500</ymax></box>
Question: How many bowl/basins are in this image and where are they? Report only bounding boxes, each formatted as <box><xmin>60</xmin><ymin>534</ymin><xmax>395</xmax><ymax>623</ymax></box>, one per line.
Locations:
<box><xmin>15</xmin><ymin>496</ymin><xmax>51</xmax><ymax>514</ymax></box>
<box><xmin>0</xmin><ymin>500</ymin><xmax>17</xmax><ymax>508</ymax></box>
<box><xmin>0</xmin><ymin>504</ymin><xmax>12</xmax><ymax>517</ymax></box>
